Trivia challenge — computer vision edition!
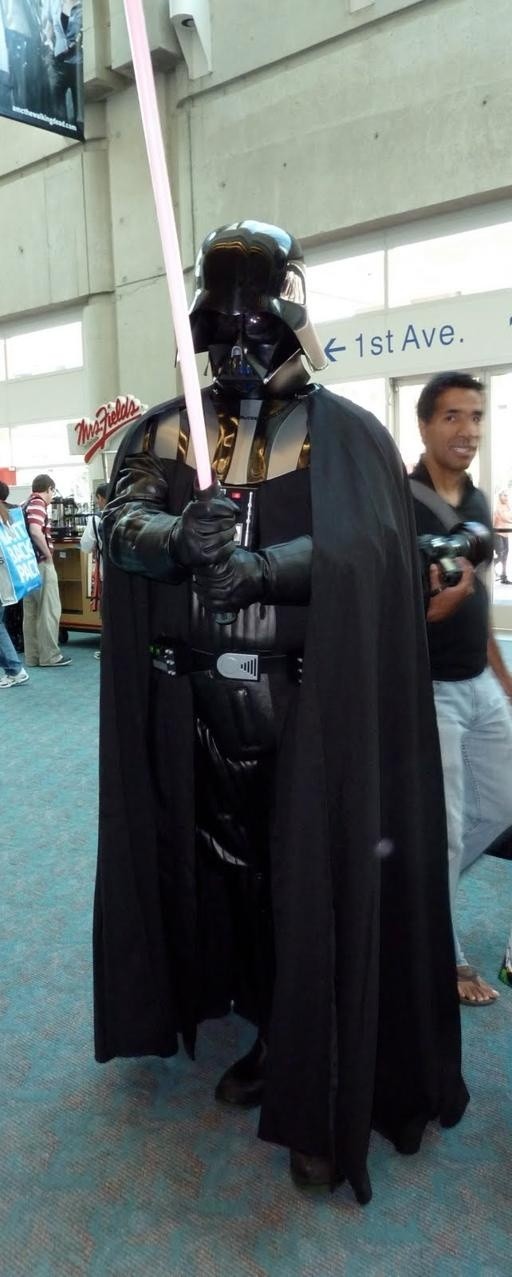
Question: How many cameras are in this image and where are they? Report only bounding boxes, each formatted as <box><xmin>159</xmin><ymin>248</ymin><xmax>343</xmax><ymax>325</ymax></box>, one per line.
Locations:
<box><xmin>418</xmin><ymin>522</ymin><xmax>491</xmax><ymax>587</ymax></box>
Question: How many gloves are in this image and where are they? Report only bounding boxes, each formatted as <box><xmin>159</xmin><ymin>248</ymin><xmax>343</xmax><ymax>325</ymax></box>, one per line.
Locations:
<box><xmin>136</xmin><ymin>498</ymin><xmax>241</xmax><ymax>575</ymax></box>
<box><xmin>194</xmin><ymin>535</ymin><xmax>314</xmax><ymax>614</ymax></box>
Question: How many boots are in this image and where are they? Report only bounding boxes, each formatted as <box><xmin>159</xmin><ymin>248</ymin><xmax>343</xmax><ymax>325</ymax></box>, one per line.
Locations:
<box><xmin>500</xmin><ymin>575</ymin><xmax>512</xmax><ymax>584</ymax></box>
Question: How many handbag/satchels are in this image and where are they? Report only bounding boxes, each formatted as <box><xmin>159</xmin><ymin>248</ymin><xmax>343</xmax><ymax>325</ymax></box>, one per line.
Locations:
<box><xmin>0</xmin><ymin>507</ymin><xmax>41</xmax><ymax>607</ymax></box>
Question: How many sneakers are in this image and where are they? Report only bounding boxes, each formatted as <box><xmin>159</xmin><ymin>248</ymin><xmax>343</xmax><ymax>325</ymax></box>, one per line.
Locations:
<box><xmin>38</xmin><ymin>656</ymin><xmax>71</xmax><ymax>667</ymax></box>
<box><xmin>0</xmin><ymin>668</ymin><xmax>30</xmax><ymax>688</ymax></box>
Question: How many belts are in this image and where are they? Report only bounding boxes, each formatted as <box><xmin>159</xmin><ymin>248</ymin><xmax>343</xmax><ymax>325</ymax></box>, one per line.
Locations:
<box><xmin>175</xmin><ymin>648</ymin><xmax>301</xmax><ymax>681</ymax></box>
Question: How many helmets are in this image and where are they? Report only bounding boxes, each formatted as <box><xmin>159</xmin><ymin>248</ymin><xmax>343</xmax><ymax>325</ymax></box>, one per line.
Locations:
<box><xmin>174</xmin><ymin>220</ymin><xmax>330</xmax><ymax>369</ymax></box>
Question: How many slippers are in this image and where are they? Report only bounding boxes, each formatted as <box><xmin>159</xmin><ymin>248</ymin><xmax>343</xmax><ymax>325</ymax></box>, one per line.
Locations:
<box><xmin>458</xmin><ymin>970</ymin><xmax>497</xmax><ymax>1006</ymax></box>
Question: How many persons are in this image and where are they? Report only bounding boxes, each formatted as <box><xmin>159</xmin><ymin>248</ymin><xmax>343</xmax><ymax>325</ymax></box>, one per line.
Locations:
<box><xmin>0</xmin><ymin>482</ymin><xmax>30</xmax><ymax>688</ymax></box>
<box><xmin>18</xmin><ymin>474</ymin><xmax>72</xmax><ymax>667</ymax></box>
<box><xmin>79</xmin><ymin>485</ymin><xmax>116</xmax><ymax>662</ymax></box>
<box><xmin>407</xmin><ymin>371</ymin><xmax>512</xmax><ymax>1004</ymax></box>
<box><xmin>493</xmin><ymin>490</ymin><xmax>512</xmax><ymax>583</ymax></box>
<box><xmin>89</xmin><ymin>219</ymin><xmax>469</xmax><ymax>1206</ymax></box>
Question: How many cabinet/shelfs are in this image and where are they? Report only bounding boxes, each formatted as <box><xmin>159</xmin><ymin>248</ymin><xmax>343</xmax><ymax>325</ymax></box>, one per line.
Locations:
<box><xmin>49</xmin><ymin>535</ymin><xmax>103</xmax><ymax>643</ymax></box>
